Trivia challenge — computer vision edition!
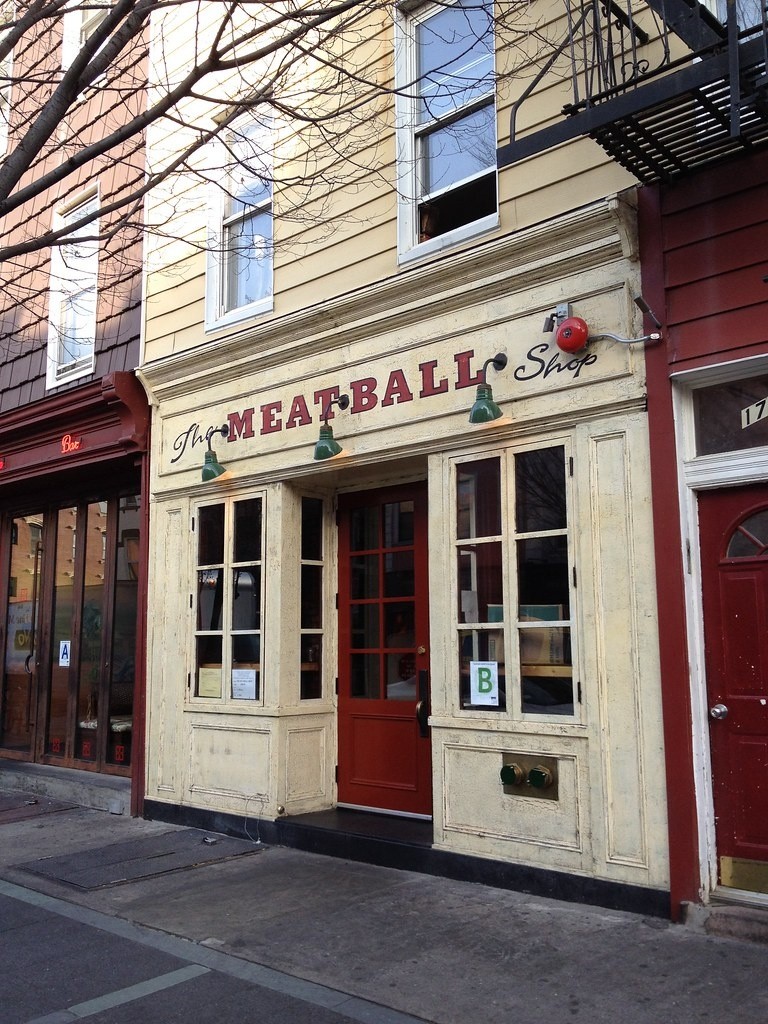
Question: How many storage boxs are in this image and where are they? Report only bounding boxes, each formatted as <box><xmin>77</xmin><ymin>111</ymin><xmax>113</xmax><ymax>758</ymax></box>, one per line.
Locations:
<box><xmin>486</xmin><ymin>604</ymin><xmax>564</xmax><ymax>664</ymax></box>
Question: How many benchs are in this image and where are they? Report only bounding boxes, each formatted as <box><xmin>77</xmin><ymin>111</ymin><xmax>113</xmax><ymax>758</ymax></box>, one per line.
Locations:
<box><xmin>77</xmin><ymin>682</ymin><xmax>134</xmax><ymax>765</ymax></box>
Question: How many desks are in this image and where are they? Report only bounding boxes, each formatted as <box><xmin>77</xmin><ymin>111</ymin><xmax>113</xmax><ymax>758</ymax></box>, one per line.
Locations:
<box><xmin>203</xmin><ymin>662</ymin><xmax>322</xmax><ymax>683</ymax></box>
<box><xmin>459</xmin><ymin>661</ymin><xmax>572</xmax><ymax>678</ymax></box>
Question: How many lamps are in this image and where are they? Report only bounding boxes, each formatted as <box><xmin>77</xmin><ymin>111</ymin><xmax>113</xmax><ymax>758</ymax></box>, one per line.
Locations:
<box><xmin>467</xmin><ymin>353</ymin><xmax>508</xmax><ymax>424</ymax></box>
<box><xmin>313</xmin><ymin>394</ymin><xmax>350</xmax><ymax>461</ymax></box>
<box><xmin>201</xmin><ymin>423</ymin><xmax>229</xmax><ymax>482</ymax></box>
